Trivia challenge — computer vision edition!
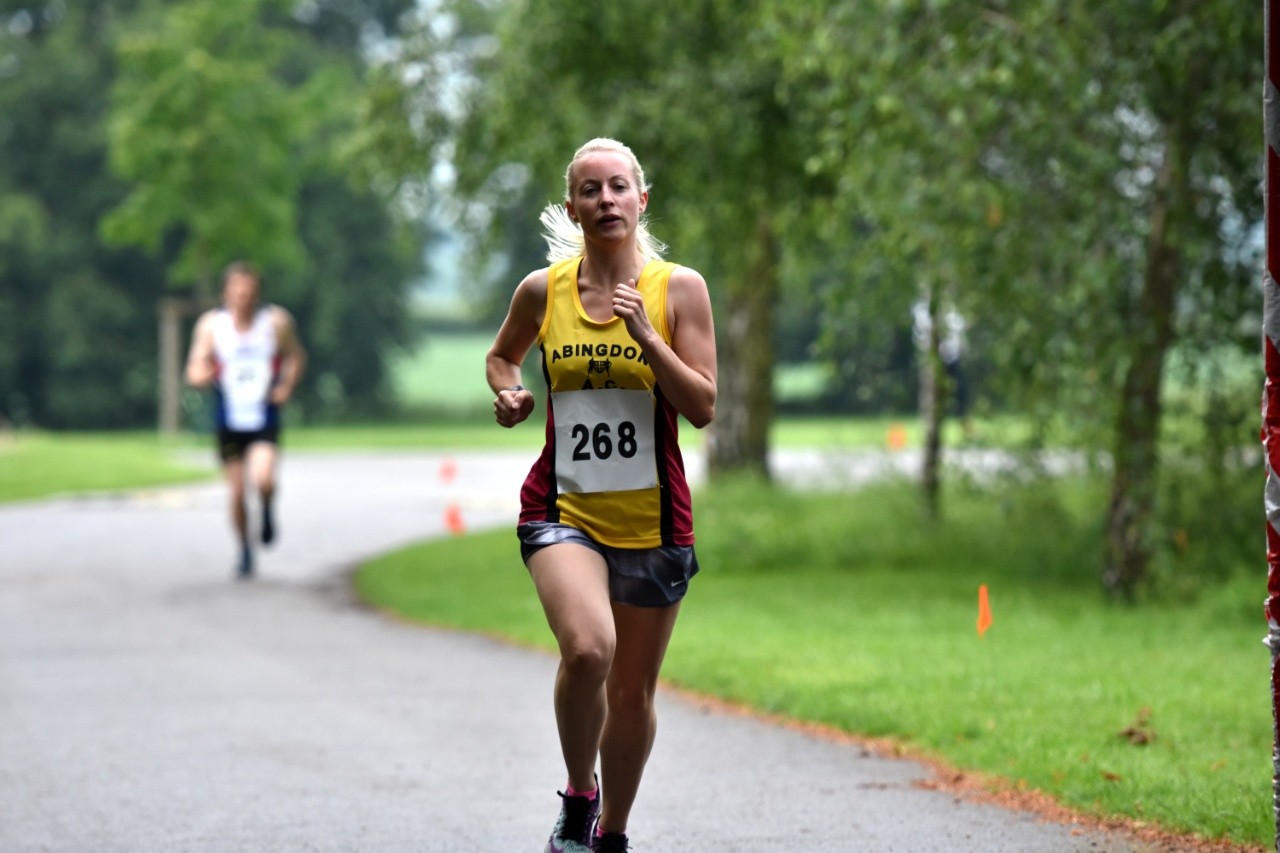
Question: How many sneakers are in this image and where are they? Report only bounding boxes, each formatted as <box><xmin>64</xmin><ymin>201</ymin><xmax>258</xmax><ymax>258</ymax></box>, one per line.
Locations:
<box><xmin>543</xmin><ymin>772</ymin><xmax>601</xmax><ymax>853</ymax></box>
<box><xmin>592</xmin><ymin>816</ymin><xmax>630</xmax><ymax>853</ymax></box>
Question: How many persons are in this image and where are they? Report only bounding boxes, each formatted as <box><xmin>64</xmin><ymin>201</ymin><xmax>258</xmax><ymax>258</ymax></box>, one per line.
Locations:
<box><xmin>487</xmin><ymin>139</ymin><xmax>715</xmax><ymax>853</ymax></box>
<box><xmin>182</xmin><ymin>260</ymin><xmax>303</xmax><ymax>576</ymax></box>
